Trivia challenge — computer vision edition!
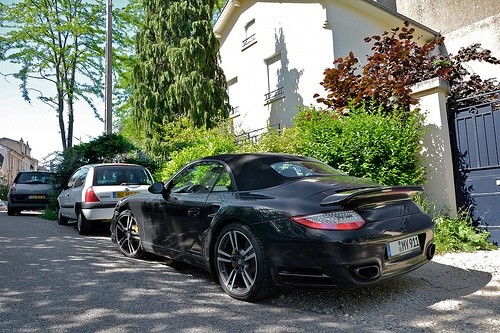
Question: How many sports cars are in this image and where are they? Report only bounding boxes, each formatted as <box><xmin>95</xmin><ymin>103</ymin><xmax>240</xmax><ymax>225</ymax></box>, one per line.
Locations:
<box><xmin>110</xmin><ymin>153</ymin><xmax>436</xmax><ymax>301</ymax></box>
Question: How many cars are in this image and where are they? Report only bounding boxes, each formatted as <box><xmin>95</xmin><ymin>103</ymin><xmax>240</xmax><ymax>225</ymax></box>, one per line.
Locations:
<box><xmin>57</xmin><ymin>162</ymin><xmax>156</xmax><ymax>234</ymax></box>
<box><xmin>6</xmin><ymin>170</ymin><xmax>63</xmax><ymax>216</ymax></box>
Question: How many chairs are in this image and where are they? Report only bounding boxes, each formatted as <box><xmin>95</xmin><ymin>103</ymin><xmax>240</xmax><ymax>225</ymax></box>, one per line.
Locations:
<box><xmin>281</xmin><ymin>168</ymin><xmax>297</xmax><ymax>177</ymax></box>
